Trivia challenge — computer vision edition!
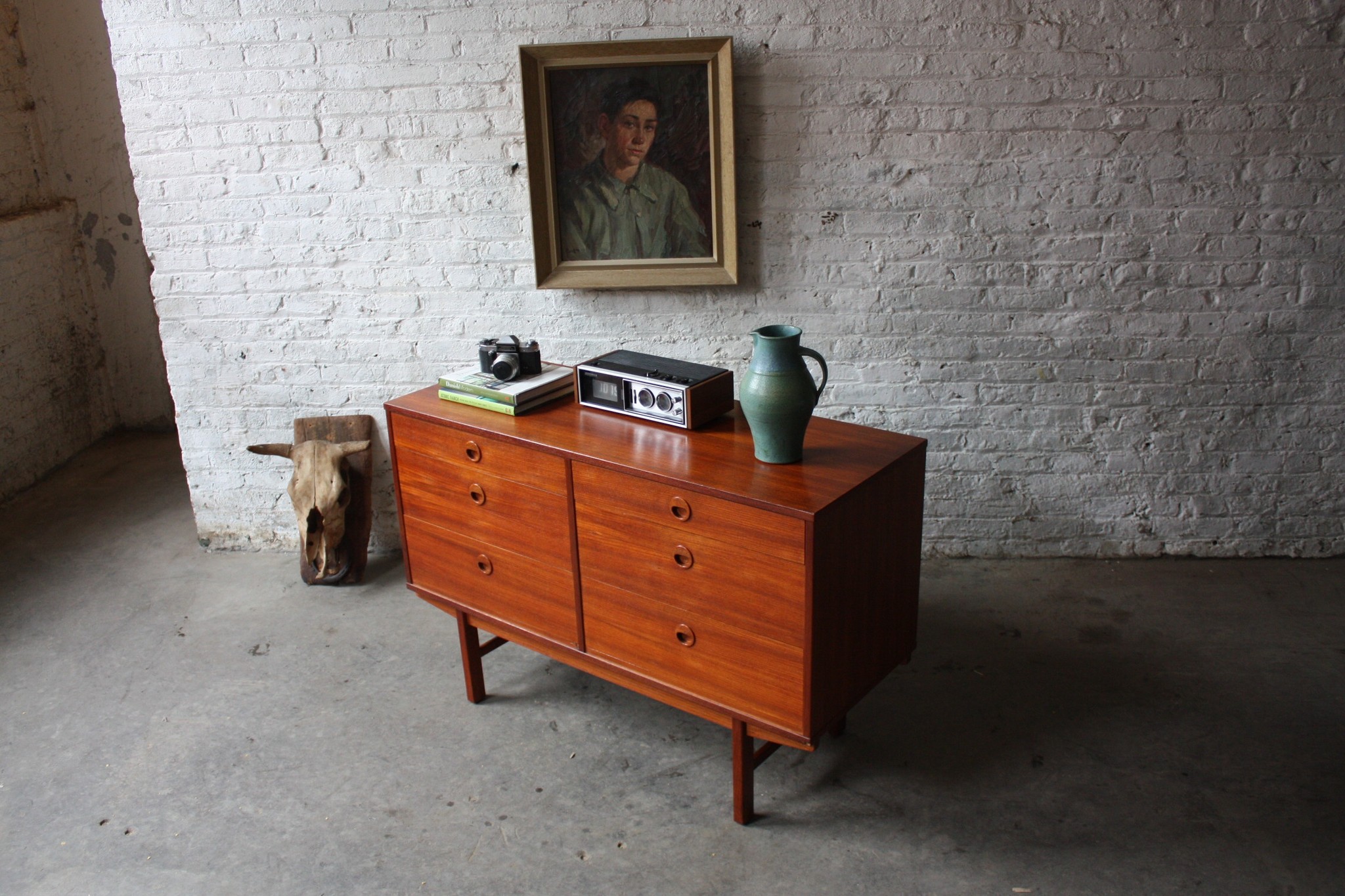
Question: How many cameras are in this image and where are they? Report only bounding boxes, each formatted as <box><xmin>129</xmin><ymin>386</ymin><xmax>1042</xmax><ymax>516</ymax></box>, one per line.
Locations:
<box><xmin>478</xmin><ymin>335</ymin><xmax>542</xmax><ymax>382</ymax></box>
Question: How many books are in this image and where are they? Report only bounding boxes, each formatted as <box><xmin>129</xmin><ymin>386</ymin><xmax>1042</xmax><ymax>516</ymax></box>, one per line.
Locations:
<box><xmin>437</xmin><ymin>362</ymin><xmax>575</xmax><ymax>417</ymax></box>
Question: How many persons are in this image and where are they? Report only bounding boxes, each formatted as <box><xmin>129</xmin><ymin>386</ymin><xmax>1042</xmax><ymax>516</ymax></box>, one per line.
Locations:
<box><xmin>559</xmin><ymin>80</ymin><xmax>709</xmax><ymax>260</ymax></box>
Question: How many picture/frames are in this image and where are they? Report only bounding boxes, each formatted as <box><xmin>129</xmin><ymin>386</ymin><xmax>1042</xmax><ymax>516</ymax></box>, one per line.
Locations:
<box><xmin>518</xmin><ymin>35</ymin><xmax>738</xmax><ymax>289</ymax></box>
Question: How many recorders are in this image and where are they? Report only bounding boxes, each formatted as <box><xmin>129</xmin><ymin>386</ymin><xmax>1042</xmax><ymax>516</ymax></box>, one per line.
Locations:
<box><xmin>573</xmin><ymin>349</ymin><xmax>736</xmax><ymax>428</ymax></box>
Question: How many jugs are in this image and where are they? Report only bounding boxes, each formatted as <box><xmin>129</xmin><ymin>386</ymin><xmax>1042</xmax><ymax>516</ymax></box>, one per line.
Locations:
<box><xmin>738</xmin><ymin>323</ymin><xmax>828</xmax><ymax>465</ymax></box>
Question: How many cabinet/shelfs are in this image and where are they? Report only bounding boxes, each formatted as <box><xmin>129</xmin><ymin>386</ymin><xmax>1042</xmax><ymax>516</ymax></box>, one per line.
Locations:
<box><xmin>382</xmin><ymin>361</ymin><xmax>928</xmax><ymax>824</ymax></box>
